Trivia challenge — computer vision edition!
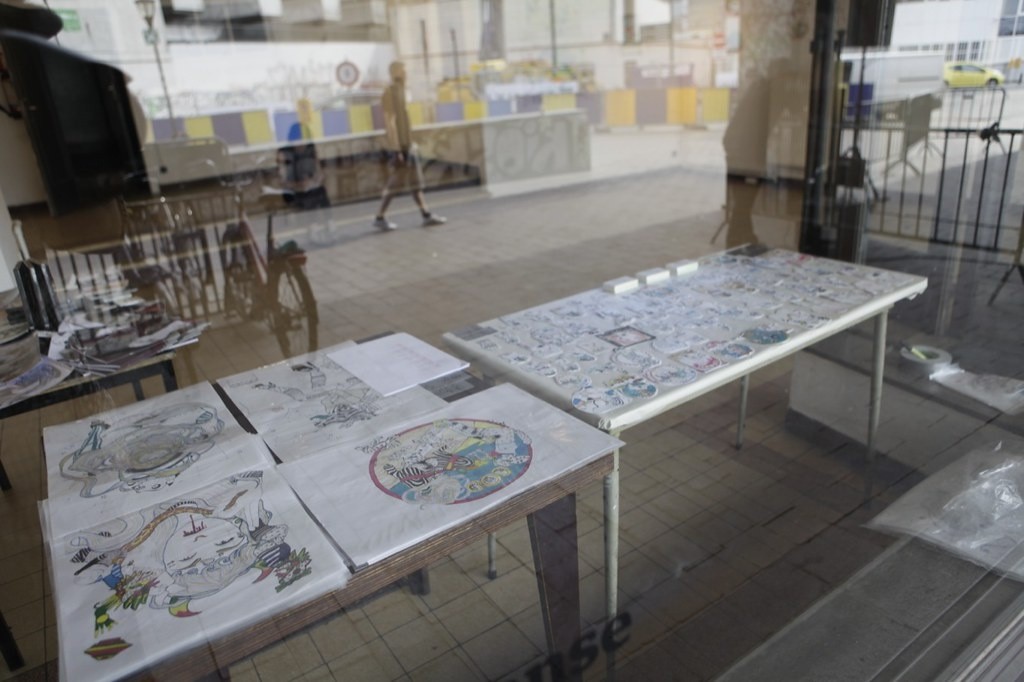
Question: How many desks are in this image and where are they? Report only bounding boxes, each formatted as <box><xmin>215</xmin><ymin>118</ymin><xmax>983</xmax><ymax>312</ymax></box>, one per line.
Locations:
<box><xmin>38</xmin><ymin>330</ymin><xmax>614</xmax><ymax>682</ymax></box>
<box><xmin>0</xmin><ymin>259</ymin><xmax>177</xmax><ymax>672</ymax></box>
<box><xmin>440</xmin><ymin>242</ymin><xmax>928</xmax><ymax>682</ymax></box>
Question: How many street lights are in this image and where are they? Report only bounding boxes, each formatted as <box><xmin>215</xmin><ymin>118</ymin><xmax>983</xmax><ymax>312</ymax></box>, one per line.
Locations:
<box><xmin>134</xmin><ymin>1</ymin><xmax>183</xmax><ymax>139</ymax></box>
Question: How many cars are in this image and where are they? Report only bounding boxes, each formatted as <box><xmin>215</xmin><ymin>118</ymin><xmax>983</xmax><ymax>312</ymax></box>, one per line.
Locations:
<box><xmin>942</xmin><ymin>60</ymin><xmax>1007</xmax><ymax>91</ymax></box>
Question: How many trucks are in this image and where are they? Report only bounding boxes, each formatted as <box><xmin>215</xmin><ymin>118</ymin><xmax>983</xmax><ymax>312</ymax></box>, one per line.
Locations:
<box><xmin>835</xmin><ymin>51</ymin><xmax>946</xmax><ymax>124</ymax></box>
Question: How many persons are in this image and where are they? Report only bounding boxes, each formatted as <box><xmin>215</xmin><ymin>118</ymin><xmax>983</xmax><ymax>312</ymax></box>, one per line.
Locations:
<box><xmin>722</xmin><ymin>58</ymin><xmax>795</xmax><ymax>258</ymax></box>
<box><xmin>279</xmin><ymin>123</ymin><xmax>333</xmax><ymax>212</ymax></box>
<box><xmin>373</xmin><ymin>62</ymin><xmax>447</xmax><ymax>231</ymax></box>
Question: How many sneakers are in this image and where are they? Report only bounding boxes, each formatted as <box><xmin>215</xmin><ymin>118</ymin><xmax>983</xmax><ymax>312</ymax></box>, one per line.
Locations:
<box><xmin>420</xmin><ymin>214</ymin><xmax>447</xmax><ymax>227</ymax></box>
<box><xmin>372</xmin><ymin>218</ymin><xmax>396</xmax><ymax>231</ymax></box>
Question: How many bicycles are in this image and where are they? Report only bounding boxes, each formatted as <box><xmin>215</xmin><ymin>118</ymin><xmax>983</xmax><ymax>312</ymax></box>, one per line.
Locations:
<box><xmin>188</xmin><ymin>150</ymin><xmax>322</xmax><ymax>363</ymax></box>
